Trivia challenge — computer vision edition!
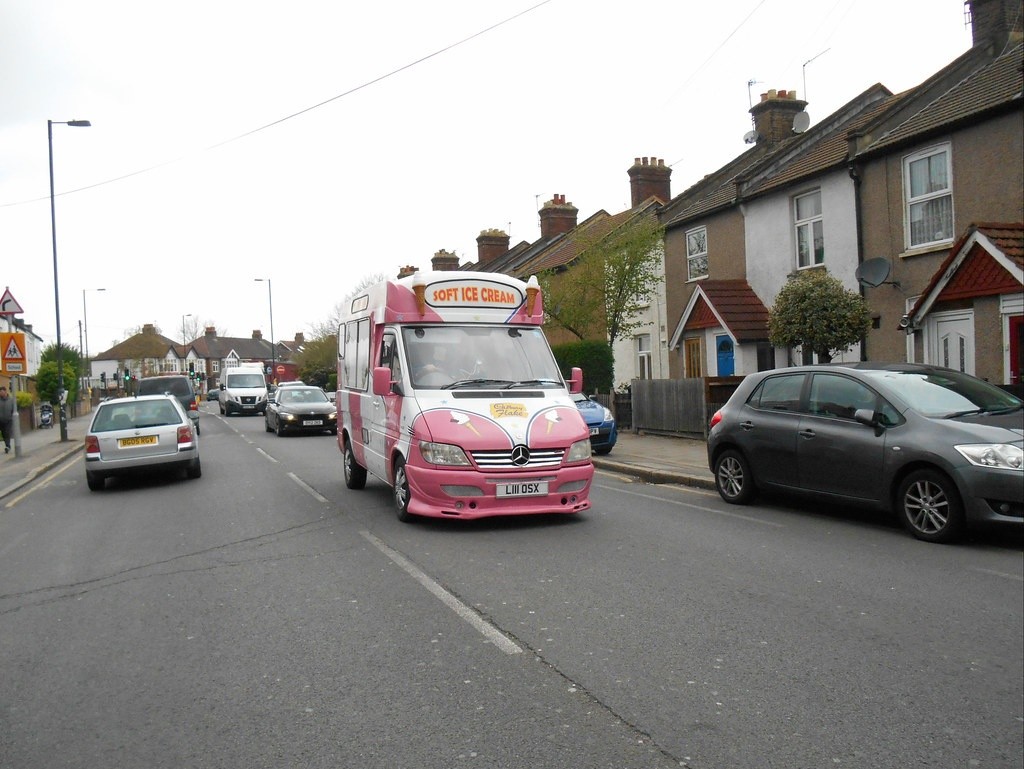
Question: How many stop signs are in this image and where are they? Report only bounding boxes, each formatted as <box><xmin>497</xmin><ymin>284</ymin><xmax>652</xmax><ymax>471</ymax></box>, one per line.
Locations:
<box><xmin>278</xmin><ymin>367</ymin><xmax>285</xmax><ymax>375</ymax></box>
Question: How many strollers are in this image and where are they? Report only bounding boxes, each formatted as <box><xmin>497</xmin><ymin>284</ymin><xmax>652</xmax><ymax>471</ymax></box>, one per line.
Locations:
<box><xmin>38</xmin><ymin>405</ymin><xmax>53</xmax><ymax>429</ymax></box>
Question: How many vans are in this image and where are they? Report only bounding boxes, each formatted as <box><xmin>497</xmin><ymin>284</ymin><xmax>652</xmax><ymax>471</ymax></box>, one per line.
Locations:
<box><xmin>333</xmin><ymin>269</ymin><xmax>596</xmax><ymax>524</ymax></box>
<box><xmin>218</xmin><ymin>368</ymin><xmax>269</xmax><ymax>416</ymax></box>
<box><xmin>138</xmin><ymin>375</ymin><xmax>201</xmax><ymax>436</ymax></box>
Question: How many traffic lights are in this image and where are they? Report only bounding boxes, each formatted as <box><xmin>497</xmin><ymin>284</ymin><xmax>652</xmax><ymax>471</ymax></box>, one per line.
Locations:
<box><xmin>100</xmin><ymin>373</ymin><xmax>105</xmax><ymax>382</ymax></box>
<box><xmin>197</xmin><ymin>373</ymin><xmax>199</xmax><ymax>381</ymax></box>
<box><xmin>124</xmin><ymin>367</ymin><xmax>130</xmax><ymax>380</ymax></box>
<box><xmin>189</xmin><ymin>362</ymin><xmax>195</xmax><ymax>376</ymax></box>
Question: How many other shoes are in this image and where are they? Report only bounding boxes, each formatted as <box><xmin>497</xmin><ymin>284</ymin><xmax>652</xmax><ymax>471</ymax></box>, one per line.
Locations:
<box><xmin>5</xmin><ymin>447</ymin><xmax>9</xmax><ymax>453</ymax></box>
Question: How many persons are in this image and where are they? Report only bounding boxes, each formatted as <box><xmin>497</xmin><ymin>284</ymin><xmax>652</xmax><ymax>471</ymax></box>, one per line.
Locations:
<box><xmin>0</xmin><ymin>386</ymin><xmax>13</xmax><ymax>453</ymax></box>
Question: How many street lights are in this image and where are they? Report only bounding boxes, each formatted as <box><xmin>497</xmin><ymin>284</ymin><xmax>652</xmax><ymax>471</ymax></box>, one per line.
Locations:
<box><xmin>182</xmin><ymin>313</ymin><xmax>192</xmax><ymax>371</ymax></box>
<box><xmin>45</xmin><ymin>117</ymin><xmax>94</xmax><ymax>443</ymax></box>
<box><xmin>253</xmin><ymin>279</ymin><xmax>277</xmax><ymax>383</ymax></box>
<box><xmin>83</xmin><ymin>288</ymin><xmax>106</xmax><ymax>375</ymax></box>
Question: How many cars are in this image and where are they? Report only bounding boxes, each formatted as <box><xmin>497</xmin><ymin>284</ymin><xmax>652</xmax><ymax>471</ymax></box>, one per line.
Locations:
<box><xmin>570</xmin><ymin>391</ymin><xmax>617</xmax><ymax>455</ymax></box>
<box><xmin>706</xmin><ymin>359</ymin><xmax>1024</xmax><ymax>541</ymax></box>
<box><xmin>325</xmin><ymin>392</ymin><xmax>336</xmax><ymax>403</ymax></box>
<box><xmin>274</xmin><ymin>380</ymin><xmax>306</xmax><ymax>397</ymax></box>
<box><xmin>206</xmin><ymin>389</ymin><xmax>219</xmax><ymax>402</ymax></box>
<box><xmin>84</xmin><ymin>390</ymin><xmax>205</xmax><ymax>492</ymax></box>
<box><xmin>265</xmin><ymin>385</ymin><xmax>337</xmax><ymax>436</ymax></box>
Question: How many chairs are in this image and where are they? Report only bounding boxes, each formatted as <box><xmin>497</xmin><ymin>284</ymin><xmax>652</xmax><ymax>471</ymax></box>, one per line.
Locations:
<box><xmin>114</xmin><ymin>414</ymin><xmax>131</xmax><ymax>425</ymax></box>
